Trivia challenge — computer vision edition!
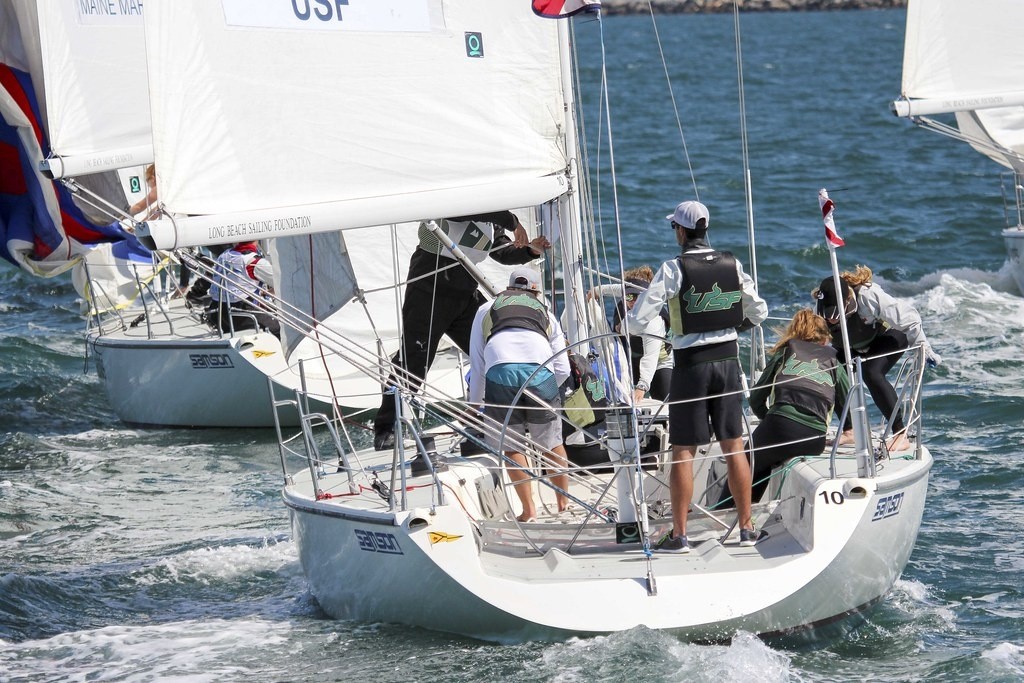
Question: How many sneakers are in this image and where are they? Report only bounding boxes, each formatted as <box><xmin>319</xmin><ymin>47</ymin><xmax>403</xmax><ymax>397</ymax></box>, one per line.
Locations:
<box><xmin>654</xmin><ymin>529</ymin><xmax>690</xmax><ymax>553</ymax></box>
<box><xmin>739</xmin><ymin>525</ymin><xmax>770</xmax><ymax>546</ymax></box>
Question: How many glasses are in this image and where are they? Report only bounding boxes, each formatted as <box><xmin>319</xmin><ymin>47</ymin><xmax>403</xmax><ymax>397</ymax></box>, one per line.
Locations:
<box><xmin>626</xmin><ymin>293</ymin><xmax>640</xmax><ymax>301</ymax></box>
<box><xmin>146</xmin><ymin>177</ymin><xmax>151</xmax><ymax>182</ymax></box>
<box><xmin>671</xmin><ymin>221</ymin><xmax>679</xmax><ymax>229</ymax></box>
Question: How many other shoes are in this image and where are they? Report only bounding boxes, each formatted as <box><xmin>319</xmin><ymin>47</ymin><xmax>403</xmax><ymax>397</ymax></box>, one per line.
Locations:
<box><xmin>374</xmin><ymin>432</ymin><xmax>396</xmax><ymax>450</ymax></box>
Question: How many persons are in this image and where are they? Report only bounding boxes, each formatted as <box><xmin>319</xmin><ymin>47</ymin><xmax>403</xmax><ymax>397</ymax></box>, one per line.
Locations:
<box><xmin>615</xmin><ymin>201</ymin><xmax>770</xmax><ymax>554</ymax></box>
<box><xmin>468</xmin><ymin>268</ymin><xmax>571</xmax><ymax>522</ymax></box>
<box><xmin>374</xmin><ymin>210</ymin><xmax>551</xmax><ymax>451</ymax></box>
<box><xmin>119</xmin><ymin>165</ymin><xmax>234</xmax><ymax>329</ymax></box>
<box><xmin>559</xmin><ymin>339</ymin><xmax>606</xmax><ymax>440</ymax></box>
<box><xmin>810</xmin><ymin>265</ymin><xmax>942</xmax><ymax>451</ymax></box>
<box><xmin>210</xmin><ymin>241</ymin><xmax>280</xmax><ymax>342</ymax></box>
<box><xmin>714</xmin><ymin>307</ymin><xmax>854</xmax><ymax>510</ymax></box>
<box><xmin>588</xmin><ymin>266</ymin><xmax>674</xmax><ymax>454</ymax></box>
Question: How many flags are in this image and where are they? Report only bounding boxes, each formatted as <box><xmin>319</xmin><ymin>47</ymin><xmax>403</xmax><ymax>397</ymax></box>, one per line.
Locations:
<box><xmin>819</xmin><ymin>192</ymin><xmax>846</xmax><ymax>250</ymax></box>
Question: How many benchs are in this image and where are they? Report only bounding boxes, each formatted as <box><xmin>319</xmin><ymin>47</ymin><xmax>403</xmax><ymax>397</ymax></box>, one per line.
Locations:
<box><xmin>748</xmin><ymin>424</ymin><xmax>919</xmax><ymax>550</ymax></box>
<box><xmin>108</xmin><ymin>282</ymin><xmax>286</xmax><ymax>374</ymax></box>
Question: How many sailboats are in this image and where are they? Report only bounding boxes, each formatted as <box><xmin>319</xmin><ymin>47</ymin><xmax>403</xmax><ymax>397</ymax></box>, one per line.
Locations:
<box><xmin>0</xmin><ymin>0</ymin><xmax>932</xmax><ymax>646</ymax></box>
<box><xmin>889</xmin><ymin>0</ymin><xmax>1024</xmax><ymax>297</ymax></box>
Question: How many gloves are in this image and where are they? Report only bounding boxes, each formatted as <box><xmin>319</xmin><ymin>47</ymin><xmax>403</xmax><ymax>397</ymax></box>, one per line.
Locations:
<box><xmin>924</xmin><ymin>347</ymin><xmax>942</xmax><ymax>367</ymax></box>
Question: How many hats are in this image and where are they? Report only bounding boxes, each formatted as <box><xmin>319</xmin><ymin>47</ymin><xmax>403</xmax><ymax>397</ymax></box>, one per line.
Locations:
<box><xmin>624</xmin><ymin>278</ymin><xmax>650</xmax><ymax>294</ymax></box>
<box><xmin>509</xmin><ymin>268</ymin><xmax>540</xmax><ymax>291</ymax></box>
<box><xmin>665</xmin><ymin>201</ymin><xmax>709</xmax><ymax>229</ymax></box>
<box><xmin>817</xmin><ymin>276</ymin><xmax>848</xmax><ymax>320</ymax></box>
